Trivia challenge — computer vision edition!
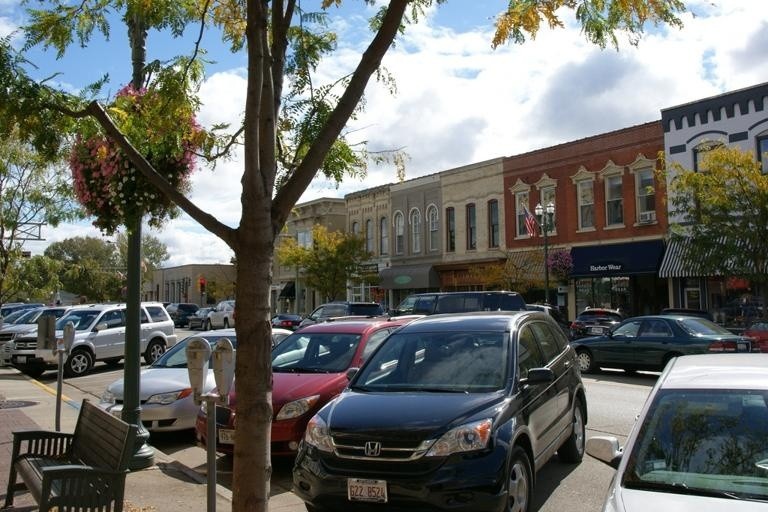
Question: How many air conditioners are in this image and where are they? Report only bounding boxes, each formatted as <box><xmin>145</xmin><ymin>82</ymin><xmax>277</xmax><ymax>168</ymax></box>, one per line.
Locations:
<box><xmin>639</xmin><ymin>211</ymin><xmax>656</xmax><ymax>222</ymax></box>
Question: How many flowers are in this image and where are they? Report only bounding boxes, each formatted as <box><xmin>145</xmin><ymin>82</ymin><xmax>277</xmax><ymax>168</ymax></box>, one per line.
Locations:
<box><xmin>70</xmin><ymin>84</ymin><xmax>205</xmax><ymax>235</ymax></box>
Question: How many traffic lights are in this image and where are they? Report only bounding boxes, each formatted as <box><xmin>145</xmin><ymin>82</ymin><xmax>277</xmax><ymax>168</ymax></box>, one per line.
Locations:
<box><xmin>200</xmin><ymin>277</ymin><xmax>205</xmax><ymax>293</ymax></box>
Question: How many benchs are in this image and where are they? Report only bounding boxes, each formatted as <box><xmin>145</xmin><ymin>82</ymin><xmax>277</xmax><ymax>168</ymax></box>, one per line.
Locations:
<box><xmin>3</xmin><ymin>399</ymin><xmax>138</xmax><ymax>512</ymax></box>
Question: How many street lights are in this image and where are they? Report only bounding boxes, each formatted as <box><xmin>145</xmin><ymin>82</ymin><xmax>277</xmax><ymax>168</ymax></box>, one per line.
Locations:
<box><xmin>531</xmin><ymin>201</ymin><xmax>559</xmax><ymax>303</ymax></box>
<box><xmin>105</xmin><ymin>238</ymin><xmax>127</xmax><ymax>250</ymax></box>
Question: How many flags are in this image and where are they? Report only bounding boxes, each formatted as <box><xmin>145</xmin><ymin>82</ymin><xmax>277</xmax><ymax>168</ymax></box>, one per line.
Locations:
<box><xmin>521</xmin><ymin>204</ymin><xmax>537</xmax><ymax>239</ymax></box>
